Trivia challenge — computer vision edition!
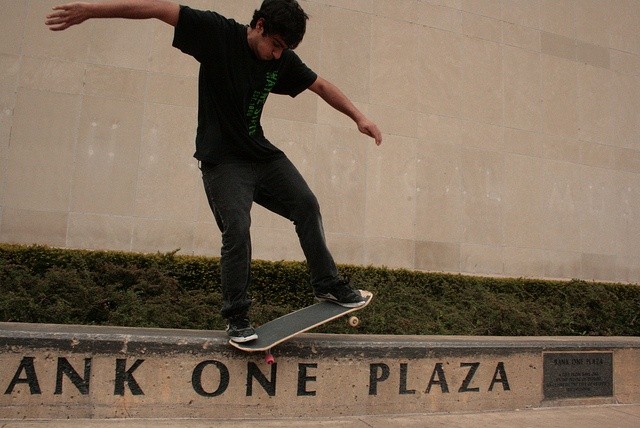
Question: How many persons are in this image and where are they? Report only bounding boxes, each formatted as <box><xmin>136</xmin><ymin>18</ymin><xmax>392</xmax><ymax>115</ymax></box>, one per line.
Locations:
<box><xmin>45</xmin><ymin>0</ymin><xmax>382</xmax><ymax>343</ymax></box>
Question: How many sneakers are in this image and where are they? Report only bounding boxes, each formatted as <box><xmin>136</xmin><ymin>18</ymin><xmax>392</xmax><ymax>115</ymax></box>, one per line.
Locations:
<box><xmin>313</xmin><ymin>279</ymin><xmax>367</xmax><ymax>308</ymax></box>
<box><xmin>225</xmin><ymin>314</ymin><xmax>258</xmax><ymax>343</ymax></box>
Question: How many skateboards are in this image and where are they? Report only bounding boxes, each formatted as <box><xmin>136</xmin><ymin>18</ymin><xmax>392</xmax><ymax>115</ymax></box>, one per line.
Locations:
<box><xmin>228</xmin><ymin>289</ymin><xmax>374</xmax><ymax>364</ymax></box>
